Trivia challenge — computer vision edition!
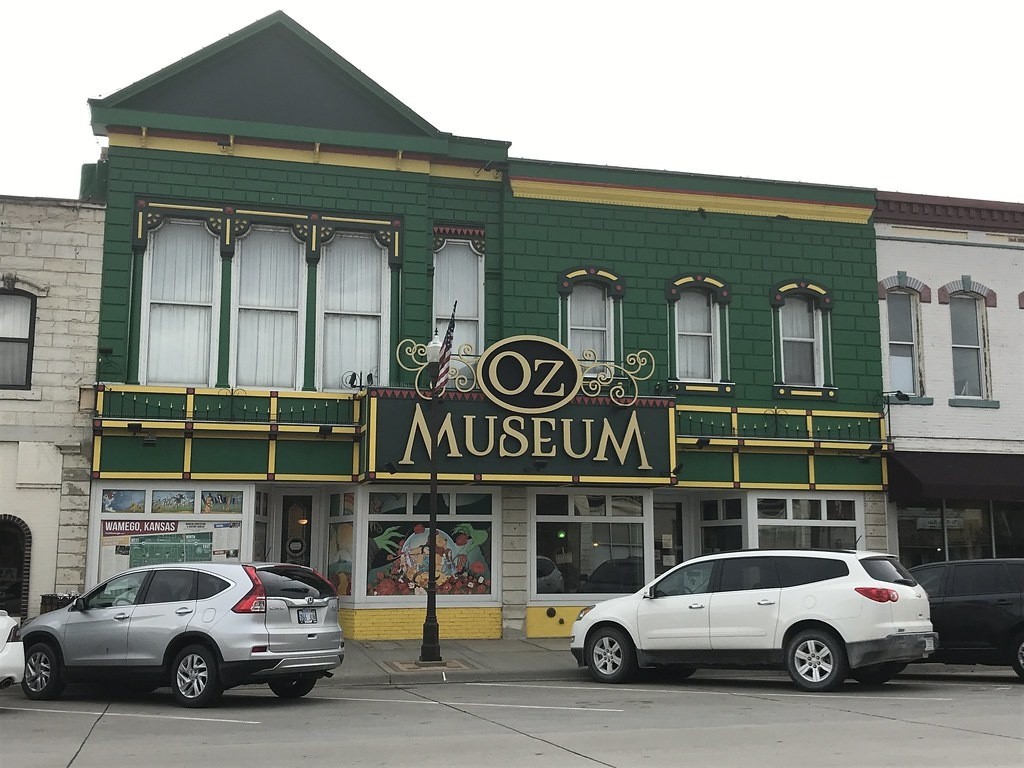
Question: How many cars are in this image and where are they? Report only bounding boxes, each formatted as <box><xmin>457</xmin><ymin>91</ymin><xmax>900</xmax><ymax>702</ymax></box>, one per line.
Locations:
<box><xmin>575</xmin><ymin>554</ymin><xmax>676</xmax><ymax>594</ymax></box>
<box><xmin>906</xmin><ymin>558</ymin><xmax>1024</xmax><ymax>681</ymax></box>
<box><xmin>16</xmin><ymin>561</ymin><xmax>345</xmax><ymax>708</ymax></box>
<box><xmin>535</xmin><ymin>553</ymin><xmax>568</xmax><ymax>593</ymax></box>
<box><xmin>570</xmin><ymin>548</ymin><xmax>939</xmax><ymax>684</ymax></box>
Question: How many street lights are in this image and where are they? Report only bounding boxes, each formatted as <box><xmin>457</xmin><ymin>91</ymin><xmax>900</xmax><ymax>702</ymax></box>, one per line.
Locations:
<box><xmin>419</xmin><ymin>329</ymin><xmax>444</xmax><ymax>662</ymax></box>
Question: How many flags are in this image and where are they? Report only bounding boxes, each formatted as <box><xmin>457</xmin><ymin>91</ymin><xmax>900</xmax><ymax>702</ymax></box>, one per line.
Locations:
<box><xmin>434</xmin><ymin>305</ymin><xmax>456</xmax><ymax>394</ymax></box>
